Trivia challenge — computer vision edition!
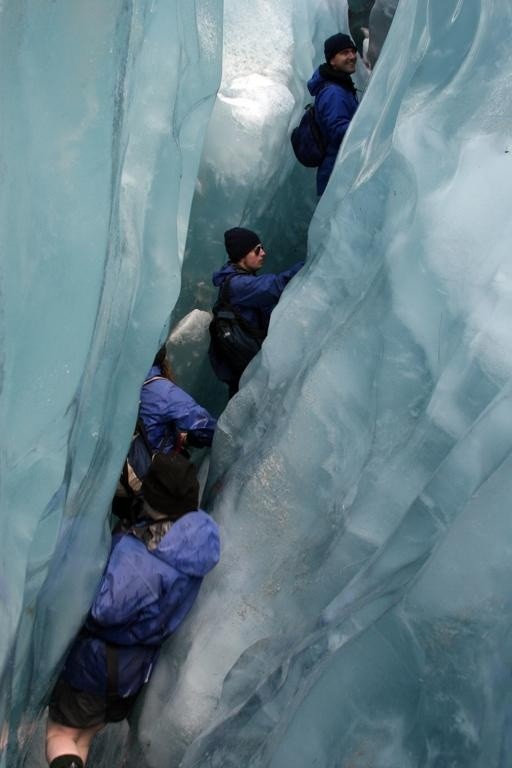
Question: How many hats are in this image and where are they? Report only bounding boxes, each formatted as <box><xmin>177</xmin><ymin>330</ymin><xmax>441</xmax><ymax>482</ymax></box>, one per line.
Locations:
<box><xmin>325</xmin><ymin>32</ymin><xmax>357</xmax><ymax>64</ymax></box>
<box><xmin>224</xmin><ymin>226</ymin><xmax>261</xmax><ymax>263</ymax></box>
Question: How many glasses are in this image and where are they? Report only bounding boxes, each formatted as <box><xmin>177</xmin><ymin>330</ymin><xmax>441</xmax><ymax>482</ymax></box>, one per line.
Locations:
<box><xmin>255</xmin><ymin>244</ymin><xmax>264</xmax><ymax>256</ymax></box>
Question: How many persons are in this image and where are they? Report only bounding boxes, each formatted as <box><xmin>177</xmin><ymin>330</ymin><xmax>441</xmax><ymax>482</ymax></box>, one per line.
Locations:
<box><xmin>45</xmin><ymin>344</ymin><xmax>221</xmax><ymax>767</ymax></box>
<box><xmin>208</xmin><ymin>227</ymin><xmax>305</xmax><ymax>401</ymax></box>
<box><xmin>307</xmin><ymin>32</ymin><xmax>377</xmax><ymax>198</ymax></box>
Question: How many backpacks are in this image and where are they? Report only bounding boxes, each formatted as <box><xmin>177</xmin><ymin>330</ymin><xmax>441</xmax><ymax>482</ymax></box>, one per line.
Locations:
<box><xmin>290</xmin><ymin>78</ymin><xmax>357</xmax><ymax>167</ymax></box>
<box><xmin>208</xmin><ymin>270</ymin><xmax>263</xmax><ymax>388</ymax></box>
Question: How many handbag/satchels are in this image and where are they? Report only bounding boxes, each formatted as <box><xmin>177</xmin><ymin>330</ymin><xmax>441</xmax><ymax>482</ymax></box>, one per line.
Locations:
<box><xmin>142</xmin><ymin>444</ymin><xmax>200</xmax><ymax>514</ymax></box>
